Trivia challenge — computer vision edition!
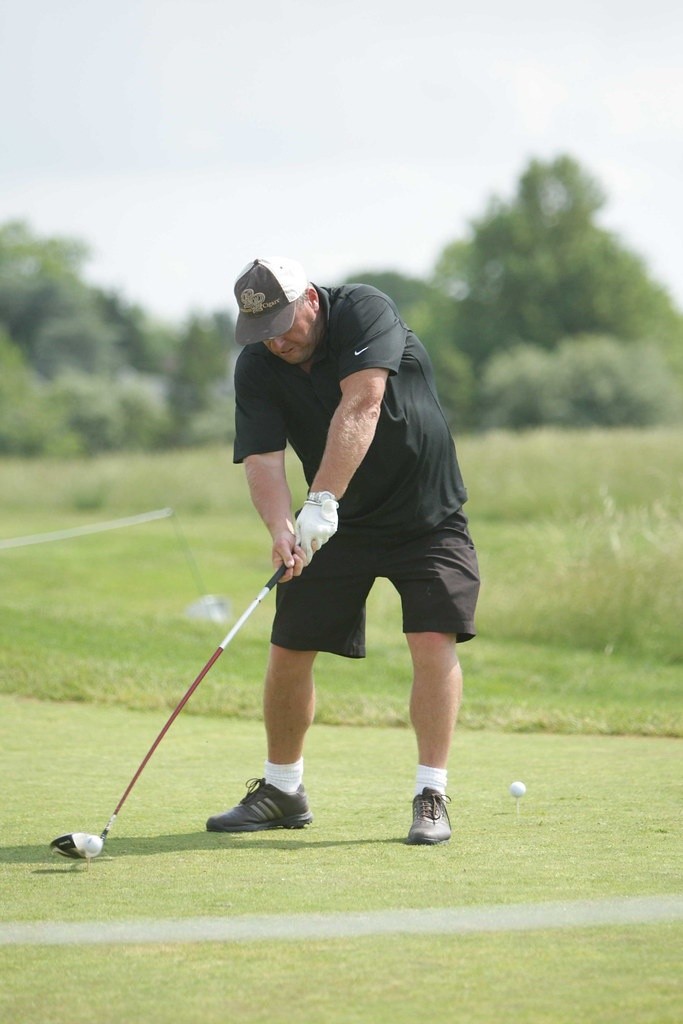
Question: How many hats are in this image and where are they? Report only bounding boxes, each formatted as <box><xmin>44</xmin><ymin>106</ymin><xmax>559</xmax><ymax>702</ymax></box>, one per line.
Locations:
<box><xmin>234</xmin><ymin>257</ymin><xmax>306</xmax><ymax>346</ymax></box>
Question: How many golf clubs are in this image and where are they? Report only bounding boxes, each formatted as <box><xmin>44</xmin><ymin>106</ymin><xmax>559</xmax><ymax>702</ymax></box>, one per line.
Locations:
<box><xmin>48</xmin><ymin>541</ymin><xmax>303</xmax><ymax>860</ymax></box>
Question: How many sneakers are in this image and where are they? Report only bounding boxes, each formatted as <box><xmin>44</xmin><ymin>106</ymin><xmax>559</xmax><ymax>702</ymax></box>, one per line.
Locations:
<box><xmin>204</xmin><ymin>777</ymin><xmax>313</xmax><ymax>833</ymax></box>
<box><xmin>407</xmin><ymin>787</ymin><xmax>452</xmax><ymax>844</ymax></box>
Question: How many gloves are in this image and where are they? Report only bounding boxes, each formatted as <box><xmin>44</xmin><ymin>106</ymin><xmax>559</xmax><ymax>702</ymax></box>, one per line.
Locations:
<box><xmin>294</xmin><ymin>499</ymin><xmax>340</xmax><ymax>567</ymax></box>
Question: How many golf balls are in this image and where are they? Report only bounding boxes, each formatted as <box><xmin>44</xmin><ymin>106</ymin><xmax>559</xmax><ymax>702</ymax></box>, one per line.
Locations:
<box><xmin>510</xmin><ymin>781</ymin><xmax>527</xmax><ymax>798</ymax></box>
<box><xmin>84</xmin><ymin>836</ymin><xmax>102</xmax><ymax>853</ymax></box>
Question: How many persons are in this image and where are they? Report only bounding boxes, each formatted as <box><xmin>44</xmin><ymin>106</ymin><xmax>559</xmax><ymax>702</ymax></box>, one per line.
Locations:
<box><xmin>206</xmin><ymin>257</ymin><xmax>480</xmax><ymax>846</ymax></box>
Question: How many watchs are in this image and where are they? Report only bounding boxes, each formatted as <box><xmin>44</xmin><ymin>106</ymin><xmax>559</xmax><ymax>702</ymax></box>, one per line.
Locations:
<box><xmin>307</xmin><ymin>490</ymin><xmax>337</xmax><ymax>503</ymax></box>
